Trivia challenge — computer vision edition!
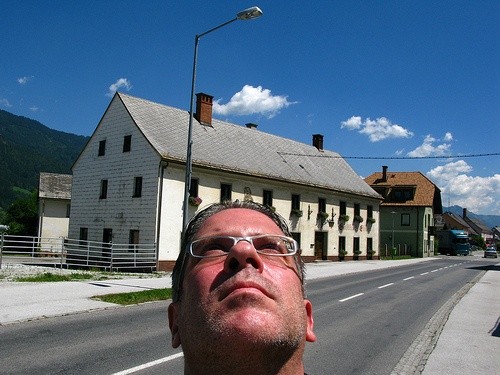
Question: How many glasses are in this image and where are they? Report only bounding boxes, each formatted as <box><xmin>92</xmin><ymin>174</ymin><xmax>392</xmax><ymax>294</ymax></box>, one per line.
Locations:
<box><xmin>175</xmin><ymin>234</ymin><xmax>304</xmax><ymax>302</ymax></box>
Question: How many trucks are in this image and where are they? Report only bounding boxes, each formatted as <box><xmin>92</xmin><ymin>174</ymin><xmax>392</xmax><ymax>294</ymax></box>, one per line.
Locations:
<box><xmin>437</xmin><ymin>230</ymin><xmax>470</xmax><ymax>256</ymax></box>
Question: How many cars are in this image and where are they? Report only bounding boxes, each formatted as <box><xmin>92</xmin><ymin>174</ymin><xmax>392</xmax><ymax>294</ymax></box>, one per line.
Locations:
<box><xmin>484</xmin><ymin>249</ymin><xmax>497</xmax><ymax>258</ymax></box>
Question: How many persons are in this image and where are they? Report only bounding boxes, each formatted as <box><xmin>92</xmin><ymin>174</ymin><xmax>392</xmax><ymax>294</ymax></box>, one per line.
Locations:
<box><xmin>166</xmin><ymin>200</ymin><xmax>316</xmax><ymax>375</ymax></box>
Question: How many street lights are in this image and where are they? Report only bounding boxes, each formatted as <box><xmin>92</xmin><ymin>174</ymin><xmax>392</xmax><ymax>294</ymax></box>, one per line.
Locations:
<box><xmin>182</xmin><ymin>6</ymin><xmax>264</xmax><ymax>233</ymax></box>
